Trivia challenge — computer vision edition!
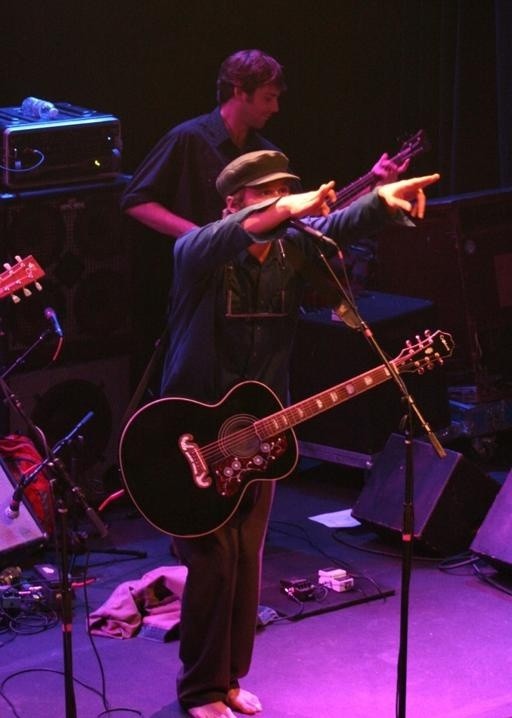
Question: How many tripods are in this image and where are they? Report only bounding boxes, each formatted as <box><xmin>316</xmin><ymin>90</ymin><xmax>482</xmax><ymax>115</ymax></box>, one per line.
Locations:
<box><xmin>1</xmin><ymin>328</ymin><xmax>148</xmax><ymax>559</ymax></box>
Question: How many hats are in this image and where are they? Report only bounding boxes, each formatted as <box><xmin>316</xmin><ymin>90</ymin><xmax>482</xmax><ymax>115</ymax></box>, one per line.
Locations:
<box><xmin>216</xmin><ymin>150</ymin><xmax>301</xmax><ymax>201</ymax></box>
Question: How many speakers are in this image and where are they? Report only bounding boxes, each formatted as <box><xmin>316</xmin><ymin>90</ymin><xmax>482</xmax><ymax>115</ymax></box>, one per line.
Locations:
<box><xmin>0</xmin><ymin>100</ymin><xmax>124</xmax><ymax>191</ymax></box>
<box><xmin>349</xmin><ymin>431</ymin><xmax>487</xmax><ymax>553</ymax></box>
<box><xmin>468</xmin><ymin>468</ymin><xmax>512</xmax><ymax>575</ymax></box>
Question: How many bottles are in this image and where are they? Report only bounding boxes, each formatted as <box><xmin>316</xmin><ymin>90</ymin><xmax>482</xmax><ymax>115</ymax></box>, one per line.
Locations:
<box><xmin>21</xmin><ymin>96</ymin><xmax>58</xmax><ymax>119</ymax></box>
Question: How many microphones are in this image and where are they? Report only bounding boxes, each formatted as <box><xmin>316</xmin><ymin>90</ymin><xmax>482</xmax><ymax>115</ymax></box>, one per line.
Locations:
<box><xmin>284</xmin><ymin>218</ymin><xmax>337</xmax><ymax>247</ymax></box>
<box><xmin>4</xmin><ymin>473</ymin><xmax>27</xmax><ymax>519</ymax></box>
<box><xmin>44</xmin><ymin>306</ymin><xmax>66</xmax><ymax>338</ymax></box>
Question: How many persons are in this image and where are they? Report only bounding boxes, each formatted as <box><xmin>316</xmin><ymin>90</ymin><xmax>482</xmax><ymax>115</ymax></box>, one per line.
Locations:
<box><xmin>116</xmin><ymin>50</ymin><xmax>414</xmax><ymax>409</ymax></box>
<box><xmin>155</xmin><ymin>146</ymin><xmax>446</xmax><ymax>718</ymax></box>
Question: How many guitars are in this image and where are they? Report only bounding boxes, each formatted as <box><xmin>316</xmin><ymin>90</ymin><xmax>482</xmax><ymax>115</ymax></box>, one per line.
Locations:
<box><xmin>119</xmin><ymin>329</ymin><xmax>455</xmax><ymax>537</ymax></box>
<box><xmin>0</xmin><ymin>252</ymin><xmax>43</xmax><ymax>303</ymax></box>
<box><xmin>327</xmin><ymin>124</ymin><xmax>427</xmax><ymax>213</ymax></box>
